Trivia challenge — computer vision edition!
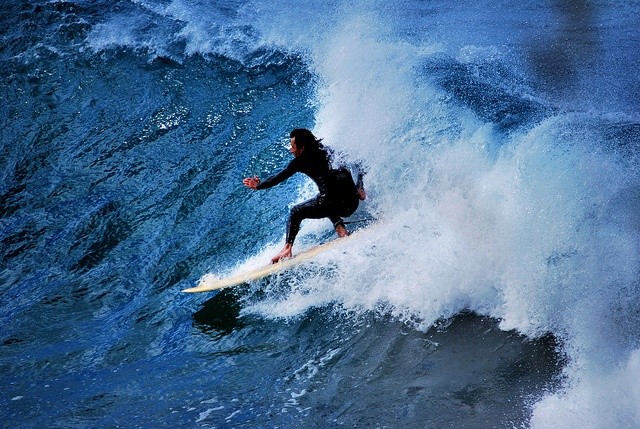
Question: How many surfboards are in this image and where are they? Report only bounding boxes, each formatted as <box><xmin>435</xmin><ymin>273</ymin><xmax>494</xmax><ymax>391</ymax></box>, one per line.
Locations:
<box><xmin>178</xmin><ymin>221</ymin><xmax>380</xmax><ymax>294</ymax></box>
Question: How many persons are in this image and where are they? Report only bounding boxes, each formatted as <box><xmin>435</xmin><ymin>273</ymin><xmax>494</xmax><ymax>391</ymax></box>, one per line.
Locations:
<box><xmin>243</xmin><ymin>128</ymin><xmax>367</xmax><ymax>263</ymax></box>
